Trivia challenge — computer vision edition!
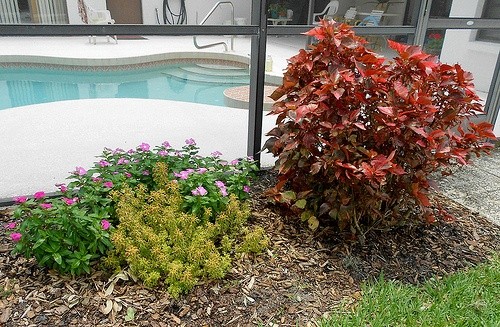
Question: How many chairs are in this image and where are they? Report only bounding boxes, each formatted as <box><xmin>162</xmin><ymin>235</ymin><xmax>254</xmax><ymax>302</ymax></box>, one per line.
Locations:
<box><xmin>353</xmin><ymin>10</ymin><xmax>387</xmax><ymax>50</ymax></box>
<box><xmin>312</xmin><ymin>0</ymin><xmax>339</xmax><ymax>25</ymax></box>
<box><xmin>87</xmin><ymin>10</ymin><xmax>118</xmax><ymax>45</ymax></box>
<box><xmin>344</xmin><ymin>7</ymin><xmax>357</xmax><ymax>23</ymax></box>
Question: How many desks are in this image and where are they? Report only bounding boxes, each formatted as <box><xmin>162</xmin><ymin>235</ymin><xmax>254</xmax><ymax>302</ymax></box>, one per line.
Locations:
<box><xmin>268</xmin><ymin>18</ymin><xmax>293</xmax><ymax>38</ymax></box>
